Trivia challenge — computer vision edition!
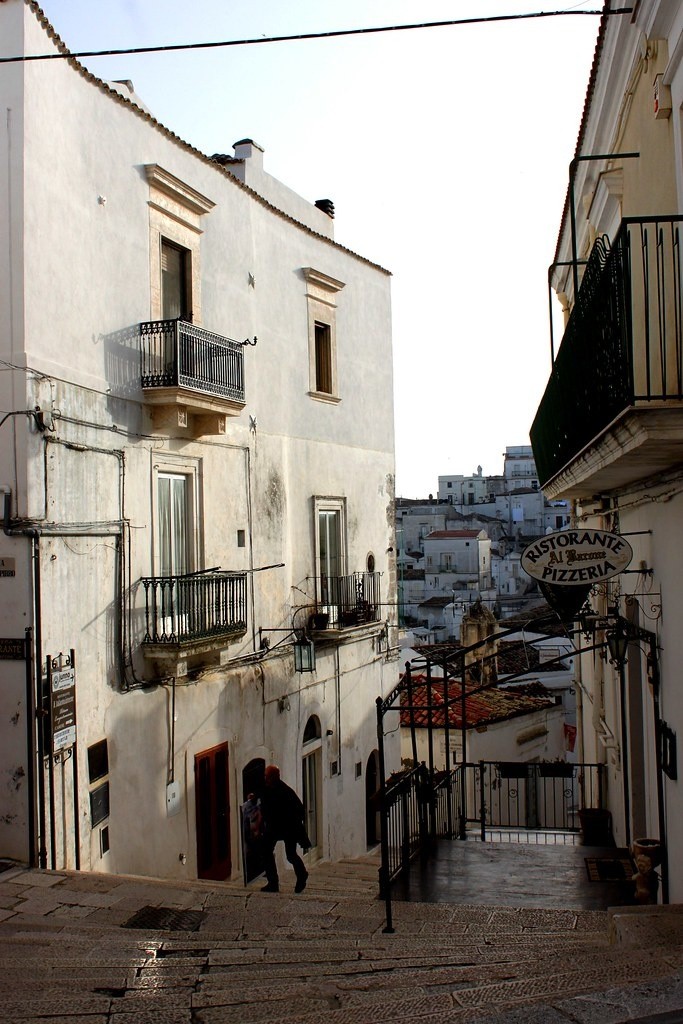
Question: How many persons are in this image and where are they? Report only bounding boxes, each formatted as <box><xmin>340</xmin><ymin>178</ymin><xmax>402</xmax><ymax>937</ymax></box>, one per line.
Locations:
<box><xmin>247</xmin><ymin>765</ymin><xmax>311</xmax><ymax>893</ymax></box>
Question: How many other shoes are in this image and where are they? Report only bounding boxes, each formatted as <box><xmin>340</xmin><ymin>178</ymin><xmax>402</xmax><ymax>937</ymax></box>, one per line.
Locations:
<box><xmin>260</xmin><ymin>882</ymin><xmax>280</xmax><ymax>893</ymax></box>
<box><xmin>294</xmin><ymin>872</ymin><xmax>308</xmax><ymax>892</ymax></box>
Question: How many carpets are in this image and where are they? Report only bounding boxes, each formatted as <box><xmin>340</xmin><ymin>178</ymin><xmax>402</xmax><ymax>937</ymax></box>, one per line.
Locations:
<box><xmin>583</xmin><ymin>857</ymin><xmax>639</xmax><ymax>882</ymax></box>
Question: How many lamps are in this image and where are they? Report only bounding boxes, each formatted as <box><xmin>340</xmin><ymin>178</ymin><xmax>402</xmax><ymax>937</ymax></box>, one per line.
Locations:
<box><xmin>605</xmin><ymin>623</ymin><xmax>629</xmax><ymax>673</ymax></box>
<box><xmin>291</xmin><ymin>627</ymin><xmax>317</xmax><ymax>674</ymax></box>
